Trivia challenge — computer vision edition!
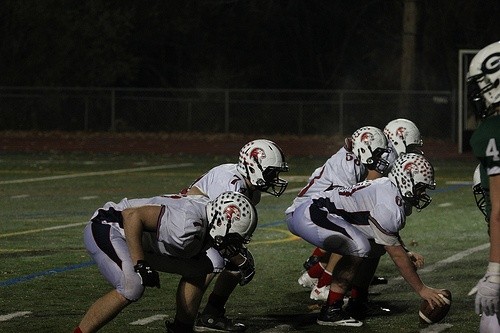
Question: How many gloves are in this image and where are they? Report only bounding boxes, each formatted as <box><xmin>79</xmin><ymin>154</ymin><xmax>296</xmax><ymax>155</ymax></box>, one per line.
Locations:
<box><xmin>467</xmin><ymin>273</ymin><xmax>500</xmax><ymax>315</ymax></box>
<box><xmin>134</xmin><ymin>259</ymin><xmax>160</xmax><ymax>290</ymax></box>
<box><xmin>238</xmin><ymin>257</ymin><xmax>255</xmax><ymax>286</ymax></box>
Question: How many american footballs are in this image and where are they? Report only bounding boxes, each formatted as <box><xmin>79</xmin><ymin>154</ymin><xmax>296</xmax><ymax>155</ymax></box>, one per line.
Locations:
<box><xmin>418</xmin><ymin>289</ymin><xmax>452</xmax><ymax>325</ymax></box>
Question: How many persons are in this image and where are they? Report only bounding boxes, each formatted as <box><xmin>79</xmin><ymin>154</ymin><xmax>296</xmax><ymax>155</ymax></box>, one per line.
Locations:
<box><xmin>182</xmin><ymin>139</ymin><xmax>288</xmax><ymax>333</ymax></box>
<box><xmin>465</xmin><ymin>40</ymin><xmax>500</xmax><ymax>333</ymax></box>
<box><xmin>72</xmin><ymin>191</ymin><xmax>259</xmax><ymax>333</ymax></box>
<box><xmin>284</xmin><ymin>119</ymin><xmax>448</xmax><ymax>327</ymax></box>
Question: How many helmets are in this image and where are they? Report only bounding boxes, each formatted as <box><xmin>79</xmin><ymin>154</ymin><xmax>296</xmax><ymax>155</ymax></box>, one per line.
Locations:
<box><xmin>472</xmin><ymin>163</ymin><xmax>489</xmax><ymax>224</ymax></box>
<box><xmin>352</xmin><ymin>126</ymin><xmax>392</xmax><ymax>177</ymax></box>
<box><xmin>466</xmin><ymin>41</ymin><xmax>500</xmax><ymax>124</ymax></box>
<box><xmin>207</xmin><ymin>191</ymin><xmax>258</xmax><ymax>259</ymax></box>
<box><xmin>237</xmin><ymin>139</ymin><xmax>288</xmax><ymax>198</ymax></box>
<box><xmin>388</xmin><ymin>153</ymin><xmax>436</xmax><ymax>210</ymax></box>
<box><xmin>384</xmin><ymin>119</ymin><xmax>425</xmax><ymax>156</ymax></box>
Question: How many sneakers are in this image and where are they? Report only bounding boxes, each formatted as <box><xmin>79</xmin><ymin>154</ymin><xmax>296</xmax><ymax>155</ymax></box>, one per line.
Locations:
<box><xmin>317</xmin><ymin>300</ymin><xmax>363</xmax><ymax>327</ymax></box>
<box><xmin>195</xmin><ymin>309</ymin><xmax>247</xmax><ymax>333</ymax></box>
<box><xmin>310</xmin><ymin>285</ymin><xmax>331</xmax><ymax>302</ymax></box>
<box><xmin>298</xmin><ymin>271</ymin><xmax>319</xmax><ymax>288</ymax></box>
<box><xmin>345</xmin><ymin>298</ymin><xmax>392</xmax><ymax>321</ymax></box>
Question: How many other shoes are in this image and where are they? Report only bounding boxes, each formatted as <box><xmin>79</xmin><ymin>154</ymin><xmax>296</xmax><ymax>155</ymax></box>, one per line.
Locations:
<box><xmin>371</xmin><ymin>276</ymin><xmax>387</xmax><ymax>285</ymax></box>
<box><xmin>165</xmin><ymin>320</ymin><xmax>195</xmax><ymax>333</ymax></box>
<box><xmin>303</xmin><ymin>255</ymin><xmax>319</xmax><ymax>271</ymax></box>
